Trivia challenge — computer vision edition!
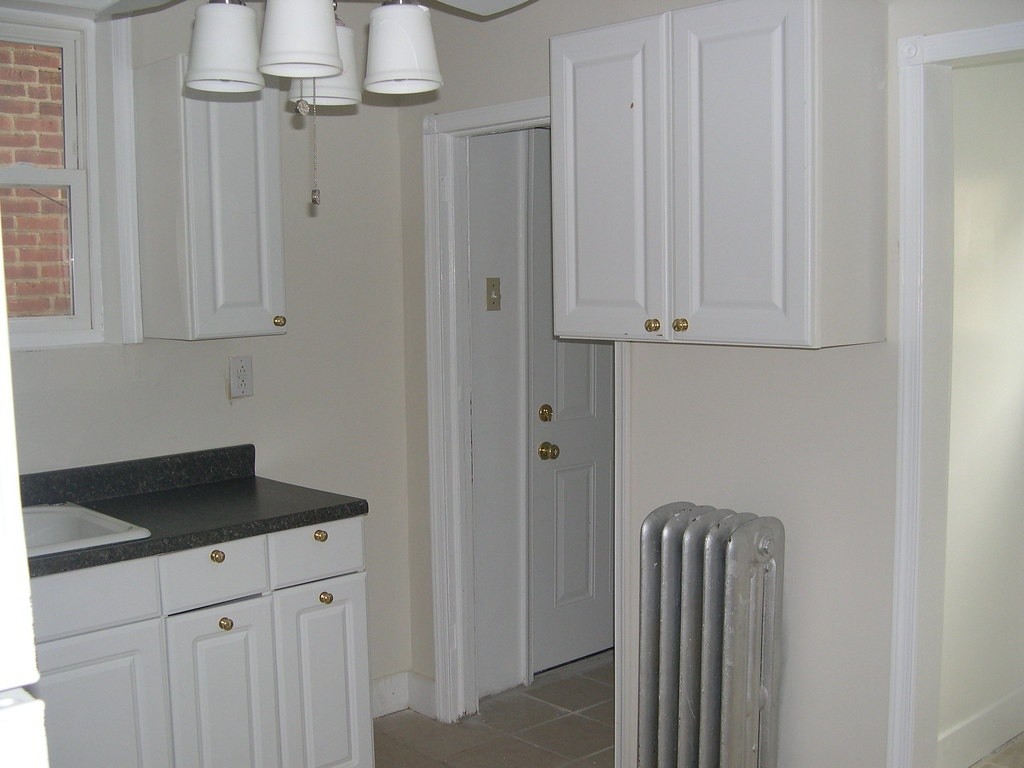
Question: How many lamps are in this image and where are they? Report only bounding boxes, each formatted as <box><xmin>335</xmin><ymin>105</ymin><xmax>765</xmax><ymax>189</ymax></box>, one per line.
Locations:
<box><xmin>185</xmin><ymin>0</ymin><xmax>444</xmax><ymax>105</ymax></box>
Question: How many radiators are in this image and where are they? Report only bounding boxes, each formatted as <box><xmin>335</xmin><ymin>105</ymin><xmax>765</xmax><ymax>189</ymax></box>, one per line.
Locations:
<box><xmin>636</xmin><ymin>501</ymin><xmax>786</xmax><ymax>768</ymax></box>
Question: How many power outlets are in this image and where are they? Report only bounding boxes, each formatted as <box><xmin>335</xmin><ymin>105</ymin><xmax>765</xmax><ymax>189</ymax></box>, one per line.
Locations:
<box><xmin>229</xmin><ymin>356</ymin><xmax>253</xmax><ymax>398</ymax></box>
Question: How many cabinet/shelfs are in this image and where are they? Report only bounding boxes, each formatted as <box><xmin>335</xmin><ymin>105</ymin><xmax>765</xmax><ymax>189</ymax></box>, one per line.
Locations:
<box><xmin>24</xmin><ymin>556</ymin><xmax>172</xmax><ymax>768</ymax></box>
<box><xmin>549</xmin><ymin>0</ymin><xmax>886</xmax><ymax>349</ymax></box>
<box><xmin>132</xmin><ymin>54</ymin><xmax>288</xmax><ymax>341</ymax></box>
<box><xmin>158</xmin><ymin>534</ymin><xmax>281</xmax><ymax>768</ymax></box>
<box><xmin>267</xmin><ymin>516</ymin><xmax>376</xmax><ymax>768</ymax></box>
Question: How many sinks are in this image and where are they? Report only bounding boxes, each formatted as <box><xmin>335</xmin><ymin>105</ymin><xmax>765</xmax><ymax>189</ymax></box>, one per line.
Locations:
<box><xmin>22</xmin><ymin>499</ymin><xmax>154</xmax><ymax>561</ymax></box>
